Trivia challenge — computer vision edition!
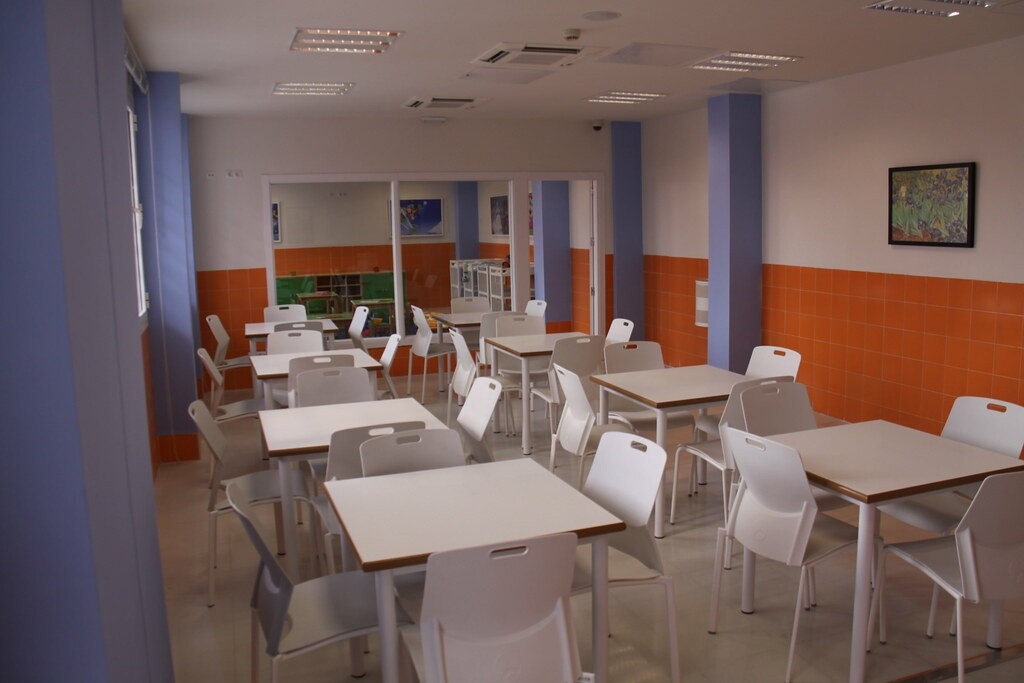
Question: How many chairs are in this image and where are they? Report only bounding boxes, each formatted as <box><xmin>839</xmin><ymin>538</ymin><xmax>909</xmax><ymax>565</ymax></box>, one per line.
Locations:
<box><xmin>181</xmin><ymin>289</ymin><xmax>1024</xmax><ymax>683</ymax></box>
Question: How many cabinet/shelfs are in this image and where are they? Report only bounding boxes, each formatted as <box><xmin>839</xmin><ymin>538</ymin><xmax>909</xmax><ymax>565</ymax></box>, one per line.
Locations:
<box><xmin>315</xmin><ymin>272</ymin><xmax>362</xmax><ymax>312</ymax></box>
<box><xmin>449</xmin><ymin>257</ymin><xmax>536</xmax><ymax>310</ymax></box>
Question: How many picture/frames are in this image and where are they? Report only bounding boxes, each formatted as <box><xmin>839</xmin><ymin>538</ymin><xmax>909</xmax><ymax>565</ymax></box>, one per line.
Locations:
<box><xmin>271</xmin><ymin>200</ymin><xmax>281</xmax><ymax>243</ymax></box>
<box><xmin>888</xmin><ymin>161</ymin><xmax>975</xmax><ymax>248</ymax></box>
<box><xmin>489</xmin><ymin>191</ymin><xmax>534</xmax><ymax>237</ymax></box>
<box><xmin>389</xmin><ymin>196</ymin><xmax>444</xmax><ymax>238</ymax></box>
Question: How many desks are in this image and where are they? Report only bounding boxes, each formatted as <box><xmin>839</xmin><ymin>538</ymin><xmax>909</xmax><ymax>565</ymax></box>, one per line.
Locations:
<box><xmin>295</xmin><ymin>290</ymin><xmax>340</xmax><ymax>313</ymax></box>
<box><xmin>308</xmin><ymin>312</ymin><xmax>354</xmax><ymax>339</ymax></box>
<box><xmin>484</xmin><ymin>331</ymin><xmax>611</xmax><ymax>454</ymax></box>
<box><xmin>742</xmin><ymin>419</ymin><xmax>1024</xmax><ymax>683</ymax></box>
<box><xmin>590</xmin><ymin>364</ymin><xmax>776</xmax><ymax>537</ymax></box>
<box><xmin>250</xmin><ymin>347</ymin><xmax>384</xmax><ymax>410</ymax></box>
<box><xmin>245</xmin><ymin>319</ymin><xmax>339</xmax><ymax>355</ymax></box>
<box><xmin>323</xmin><ymin>457</ymin><xmax>626</xmax><ymax>683</ymax></box>
<box><xmin>431</xmin><ymin>310</ymin><xmax>489</xmax><ymax>391</ymax></box>
<box><xmin>350</xmin><ymin>297</ymin><xmax>396</xmax><ymax>336</ymax></box>
<box><xmin>257</xmin><ymin>396</ymin><xmax>452</xmax><ymax>580</ymax></box>
